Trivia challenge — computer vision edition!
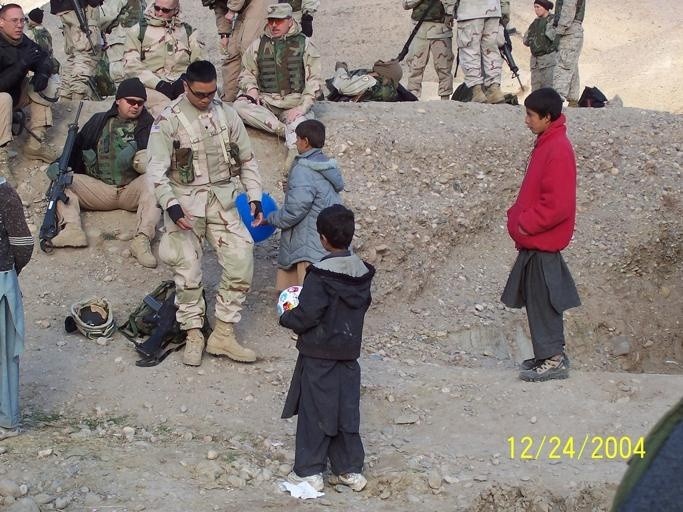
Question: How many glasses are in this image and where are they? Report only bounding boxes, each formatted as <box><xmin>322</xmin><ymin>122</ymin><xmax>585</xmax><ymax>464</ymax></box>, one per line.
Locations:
<box><xmin>121</xmin><ymin>96</ymin><xmax>144</xmax><ymax>106</ymax></box>
<box><xmin>268</xmin><ymin>18</ymin><xmax>286</xmax><ymax>24</ymax></box>
<box><xmin>154</xmin><ymin>3</ymin><xmax>175</xmax><ymax>13</ymax></box>
<box><xmin>0</xmin><ymin>15</ymin><xmax>26</xmax><ymax>28</ymax></box>
<box><xmin>186</xmin><ymin>81</ymin><xmax>218</xmax><ymax>98</ymax></box>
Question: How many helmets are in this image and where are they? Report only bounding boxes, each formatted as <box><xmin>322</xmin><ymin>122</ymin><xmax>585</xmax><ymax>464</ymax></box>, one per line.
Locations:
<box><xmin>70</xmin><ymin>294</ymin><xmax>115</xmax><ymax>340</ymax></box>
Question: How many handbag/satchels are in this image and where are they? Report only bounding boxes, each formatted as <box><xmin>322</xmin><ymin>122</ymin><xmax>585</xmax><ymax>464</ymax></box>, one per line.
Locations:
<box><xmin>577</xmin><ymin>85</ymin><xmax>608</xmax><ymax>107</ymax></box>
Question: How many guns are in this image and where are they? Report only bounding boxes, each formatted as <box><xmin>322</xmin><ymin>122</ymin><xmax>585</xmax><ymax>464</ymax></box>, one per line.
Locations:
<box><xmin>136</xmin><ymin>290</ymin><xmax>179</xmax><ymax>358</ymax></box>
<box><xmin>40</xmin><ymin>101</ymin><xmax>84</xmax><ymax>240</ymax></box>
<box><xmin>72</xmin><ymin>0</ymin><xmax>98</xmax><ymax>56</ymax></box>
<box><xmin>499</xmin><ymin>25</ymin><xmax>524</xmax><ymax>92</ymax></box>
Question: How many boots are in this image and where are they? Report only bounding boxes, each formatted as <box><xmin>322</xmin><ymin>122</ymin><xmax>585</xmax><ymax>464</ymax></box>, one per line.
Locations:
<box><xmin>206</xmin><ymin>316</ymin><xmax>257</xmax><ymax>364</ymax></box>
<box><xmin>182</xmin><ymin>326</ymin><xmax>206</xmax><ymax>366</ymax></box>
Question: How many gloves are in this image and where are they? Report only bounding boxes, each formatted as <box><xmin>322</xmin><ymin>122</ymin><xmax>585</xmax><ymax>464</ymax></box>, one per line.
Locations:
<box><xmin>46</xmin><ymin>163</ymin><xmax>65</xmax><ymax>180</ymax></box>
<box><xmin>30</xmin><ymin>73</ymin><xmax>49</xmax><ymax>92</ymax></box>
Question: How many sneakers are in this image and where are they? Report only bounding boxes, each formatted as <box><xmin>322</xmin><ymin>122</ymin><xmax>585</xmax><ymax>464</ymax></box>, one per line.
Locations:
<box><xmin>24</xmin><ymin>137</ymin><xmax>59</xmax><ymax>164</ymax></box>
<box><xmin>45</xmin><ymin>222</ymin><xmax>88</xmax><ymax>249</ymax></box>
<box><xmin>520</xmin><ymin>349</ymin><xmax>571</xmax><ymax>382</ymax></box>
<box><xmin>129</xmin><ymin>235</ymin><xmax>158</xmax><ymax>269</ymax></box>
<box><xmin>328</xmin><ymin>470</ymin><xmax>368</xmax><ymax>492</ymax></box>
<box><xmin>287</xmin><ymin>470</ymin><xmax>326</xmax><ymax>491</ymax></box>
<box><xmin>1</xmin><ymin>150</ymin><xmax>18</xmax><ymax>189</ymax></box>
<box><xmin>470</xmin><ymin>83</ymin><xmax>505</xmax><ymax>105</ymax></box>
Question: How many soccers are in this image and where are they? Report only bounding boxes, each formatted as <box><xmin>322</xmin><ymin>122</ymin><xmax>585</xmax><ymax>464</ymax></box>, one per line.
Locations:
<box><xmin>277</xmin><ymin>286</ymin><xmax>302</xmax><ymax>316</ymax></box>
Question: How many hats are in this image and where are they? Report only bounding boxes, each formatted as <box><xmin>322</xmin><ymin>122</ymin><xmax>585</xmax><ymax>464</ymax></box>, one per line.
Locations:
<box><xmin>116</xmin><ymin>78</ymin><xmax>147</xmax><ymax>101</ymax></box>
<box><xmin>535</xmin><ymin>0</ymin><xmax>553</xmax><ymax>11</ymax></box>
<box><xmin>264</xmin><ymin>2</ymin><xmax>293</xmax><ymax>19</ymax></box>
<box><xmin>28</xmin><ymin>7</ymin><xmax>44</xmax><ymax>24</ymax></box>
